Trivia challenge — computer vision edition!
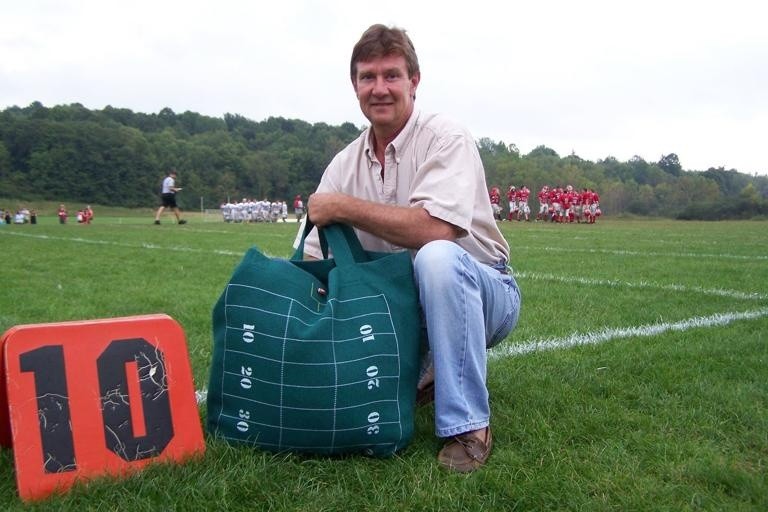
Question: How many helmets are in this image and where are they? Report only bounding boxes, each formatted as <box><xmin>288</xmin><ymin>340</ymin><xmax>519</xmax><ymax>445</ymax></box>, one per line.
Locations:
<box><xmin>584</xmin><ymin>210</ymin><xmax>590</xmax><ymax>216</ymax></box>
<box><xmin>569</xmin><ymin>212</ymin><xmax>575</xmax><ymax>217</ymax></box>
<box><xmin>492</xmin><ymin>188</ymin><xmax>498</xmax><ymax>193</ymax></box>
<box><xmin>542</xmin><ymin>186</ymin><xmax>548</xmax><ymax>192</ymax></box>
<box><xmin>509</xmin><ymin>186</ymin><xmax>514</xmax><ymax>190</ymax></box>
<box><xmin>549</xmin><ymin>208</ymin><xmax>554</xmax><ymax>214</ymax></box>
<box><xmin>568</xmin><ymin>186</ymin><xmax>572</xmax><ymax>190</ymax></box>
<box><xmin>595</xmin><ymin>209</ymin><xmax>601</xmax><ymax>216</ymax></box>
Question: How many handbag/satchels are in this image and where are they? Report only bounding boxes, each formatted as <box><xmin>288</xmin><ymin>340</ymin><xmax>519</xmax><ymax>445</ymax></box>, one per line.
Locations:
<box><xmin>208</xmin><ymin>247</ymin><xmax>422</xmax><ymax>457</ymax></box>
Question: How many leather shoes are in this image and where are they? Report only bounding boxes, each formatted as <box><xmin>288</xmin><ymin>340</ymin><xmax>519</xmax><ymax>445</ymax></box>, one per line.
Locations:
<box><xmin>414</xmin><ymin>370</ymin><xmax>434</xmax><ymax>405</ymax></box>
<box><xmin>436</xmin><ymin>426</ymin><xmax>493</xmax><ymax>473</ymax></box>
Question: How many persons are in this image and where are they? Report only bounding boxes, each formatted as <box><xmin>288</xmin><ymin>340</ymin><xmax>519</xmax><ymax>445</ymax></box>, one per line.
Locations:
<box><xmin>152</xmin><ymin>170</ymin><xmax>188</xmax><ymax>225</ymax></box>
<box><xmin>220</xmin><ymin>195</ymin><xmax>288</xmax><ymax>224</ymax></box>
<box><xmin>14</xmin><ymin>207</ymin><xmax>30</xmax><ymax>225</ymax></box>
<box><xmin>77</xmin><ymin>203</ymin><xmax>94</xmax><ymax>225</ymax></box>
<box><xmin>287</xmin><ymin>21</ymin><xmax>523</xmax><ymax>473</ymax></box>
<box><xmin>504</xmin><ymin>185</ymin><xmax>520</xmax><ymax>221</ymax></box>
<box><xmin>57</xmin><ymin>203</ymin><xmax>67</xmax><ymax>224</ymax></box>
<box><xmin>5</xmin><ymin>211</ymin><xmax>11</xmax><ymax>224</ymax></box>
<box><xmin>536</xmin><ymin>184</ymin><xmax>601</xmax><ymax>226</ymax></box>
<box><xmin>293</xmin><ymin>195</ymin><xmax>305</xmax><ymax>222</ymax></box>
<box><xmin>514</xmin><ymin>184</ymin><xmax>532</xmax><ymax>221</ymax></box>
<box><xmin>30</xmin><ymin>209</ymin><xmax>37</xmax><ymax>224</ymax></box>
<box><xmin>488</xmin><ymin>187</ymin><xmax>505</xmax><ymax>222</ymax></box>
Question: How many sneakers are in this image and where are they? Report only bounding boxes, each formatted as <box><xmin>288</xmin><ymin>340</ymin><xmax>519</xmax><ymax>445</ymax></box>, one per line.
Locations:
<box><xmin>179</xmin><ymin>220</ymin><xmax>186</xmax><ymax>224</ymax></box>
<box><xmin>154</xmin><ymin>221</ymin><xmax>161</xmax><ymax>224</ymax></box>
